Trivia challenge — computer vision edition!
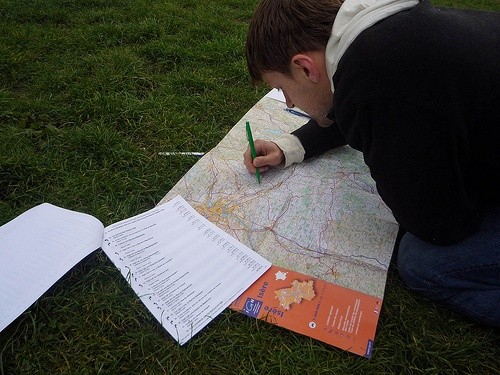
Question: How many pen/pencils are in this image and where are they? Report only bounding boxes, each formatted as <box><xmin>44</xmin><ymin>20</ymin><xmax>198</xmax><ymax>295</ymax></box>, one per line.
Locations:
<box><xmin>246</xmin><ymin>121</ymin><xmax>264</xmax><ymax>187</ymax></box>
<box><xmin>283</xmin><ymin>108</ymin><xmax>311</xmax><ymax>119</ymax></box>
<box><xmin>158</xmin><ymin>151</ymin><xmax>205</xmax><ymax>156</ymax></box>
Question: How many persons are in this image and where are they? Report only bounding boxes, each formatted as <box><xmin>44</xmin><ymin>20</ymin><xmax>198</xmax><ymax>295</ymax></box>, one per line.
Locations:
<box><xmin>243</xmin><ymin>0</ymin><xmax>500</xmax><ymax>330</ymax></box>
